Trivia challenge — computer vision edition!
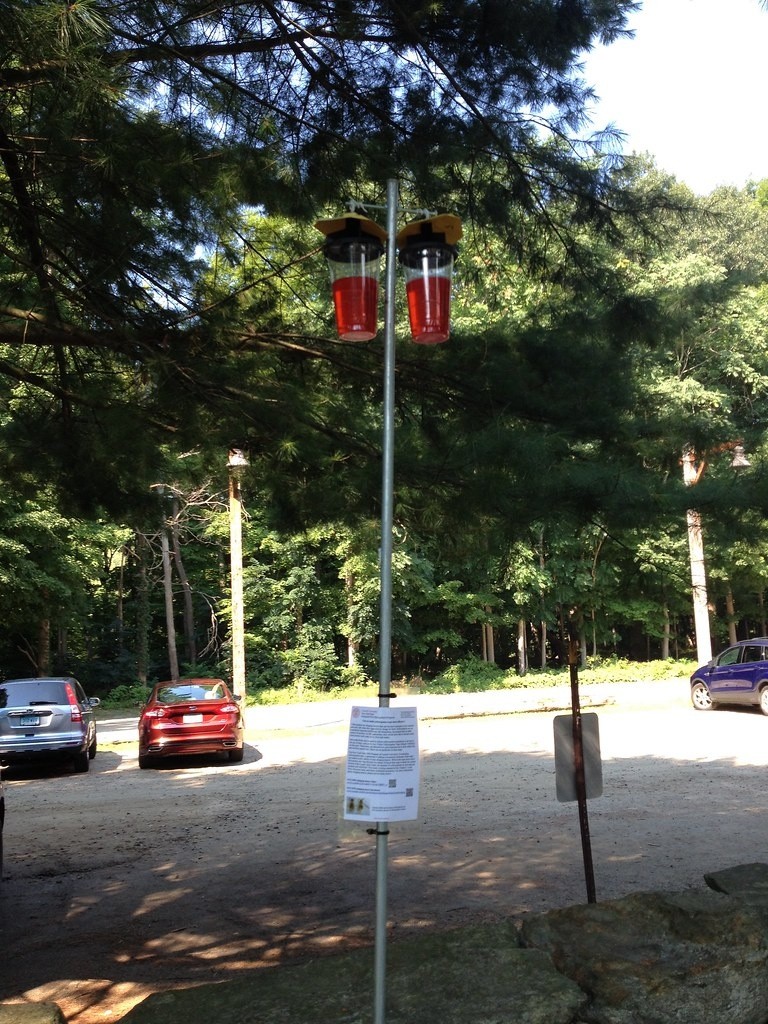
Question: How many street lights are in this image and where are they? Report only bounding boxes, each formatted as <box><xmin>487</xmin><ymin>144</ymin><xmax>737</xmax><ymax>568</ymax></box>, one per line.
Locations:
<box><xmin>314</xmin><ymin>140</ymin><xmax>464</xmax><ymax>1024</ymax></box>
<box><xmin>680</xmin><ymin>435</ymin><xmax>753</xmax><ymax>671</ymax></box>
<box><xmin>177</xmin><ymin>444</ymin><xmax>249</xmax><ymax>731</ymax></box>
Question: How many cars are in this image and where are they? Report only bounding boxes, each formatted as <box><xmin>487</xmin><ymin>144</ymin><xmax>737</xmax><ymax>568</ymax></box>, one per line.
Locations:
<box><xmin>0</xmin><ymin>677</ymin><xmax>102</xmax><ymax>773</ymax></box>
<box><xmin>131</xmin><ymin>678</ymin><xmax>246</xmax><ymax>770</ymax></box>
<box><xmin>689</xmin><ymin>635</ymin><xmax>768</xmax><ymax>717</ymax></box>
